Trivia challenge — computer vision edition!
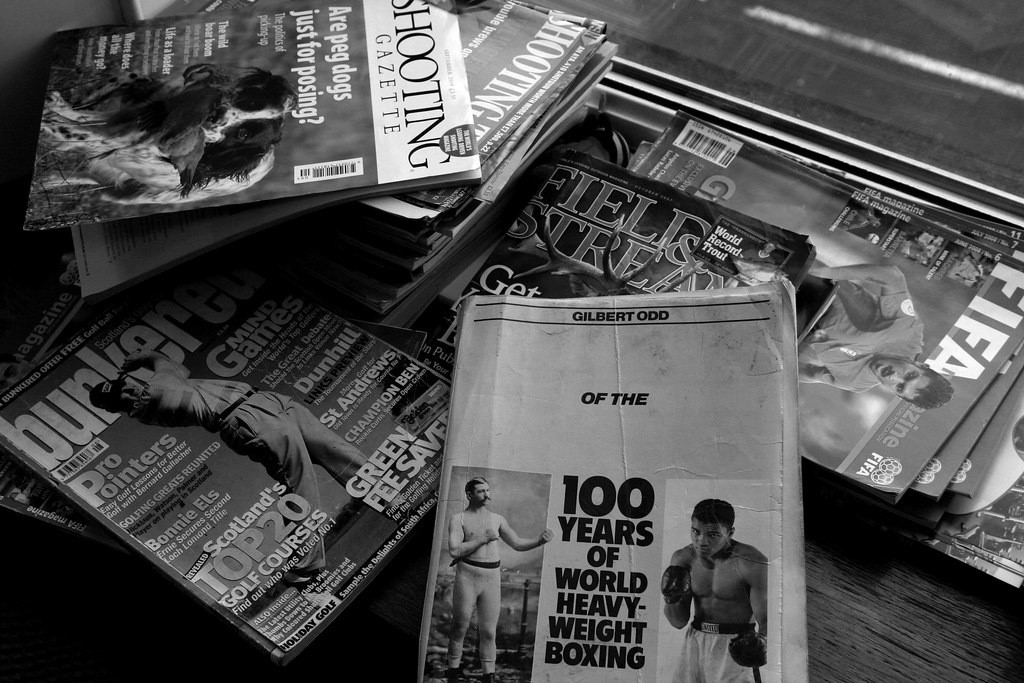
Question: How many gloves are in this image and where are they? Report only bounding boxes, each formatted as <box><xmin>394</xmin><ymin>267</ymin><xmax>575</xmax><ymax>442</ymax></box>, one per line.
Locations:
<box><xmin>661</xmin><ymin>566</ymin><xmax>691</xmax><ymax>605</ymax></box>
<box><xmin>730</xmin><ymin>634</ymin><xmax>768</xmax><ymax>667</ymax></box>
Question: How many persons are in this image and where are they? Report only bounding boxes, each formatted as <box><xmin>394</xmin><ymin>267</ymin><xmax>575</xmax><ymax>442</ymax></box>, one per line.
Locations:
<box><xmin>445</xmin><ymin>476</ymin><xmax>555</xmax><ymax>681</ymax></box>
<box><xmin>798</xmin><ymin>217</ymin><xmax>955</xmax><ymax>409</ymax></box>
<box><xmin>90</xmin><ymin>346</ymin><xmax>381</xmax><ymax>582</ymax></box>
<box><xmin>660</xmin><ymin>498</ymin><xmax>769</xmax><ymax>683</ymax></box>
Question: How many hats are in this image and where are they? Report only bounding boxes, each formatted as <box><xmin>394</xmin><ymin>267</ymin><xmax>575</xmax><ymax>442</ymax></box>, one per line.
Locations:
<box><xmin>89</xmin><ymin>376</ymin><xmax>127</xmax><ymax>412</ymax></box>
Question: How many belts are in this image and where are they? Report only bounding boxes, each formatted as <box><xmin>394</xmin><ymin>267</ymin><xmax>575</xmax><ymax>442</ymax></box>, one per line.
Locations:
<box><xmin>449</xmin><ymin>557</ymin><xmax>501</xmax><ymax>569</ymax></box>
<box><xmin>690</xmin><ymin>619</ymin><xmax>758</xmax><ymax>635</ymax></box>
<box><xmin>220</xmin><ymin>387</ymin><xmax>259</xmax><ymax>419</ymax></box>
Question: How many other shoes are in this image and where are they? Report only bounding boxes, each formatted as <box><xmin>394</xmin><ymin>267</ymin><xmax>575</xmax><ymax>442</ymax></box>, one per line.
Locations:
<box><xmin>482</xmin><ymin>672</ymin><xmax>496</xmax><ymax>683</ymax></box>
<box><xmin>282</xmin><ymin>570</ymin><xmax>328</xmax><ymax>594</ymax></box>
<box><xmin>449</xmin><ymin>668</ymin><xmax>471</xmax><ymax>683</ymax></box>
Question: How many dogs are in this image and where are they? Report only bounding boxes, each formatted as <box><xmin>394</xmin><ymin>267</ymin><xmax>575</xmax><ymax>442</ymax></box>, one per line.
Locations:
<box><xmin>39</xmin><ymin>63</ymin><xmax>299</xmax><ymax>206</ymax></box>
